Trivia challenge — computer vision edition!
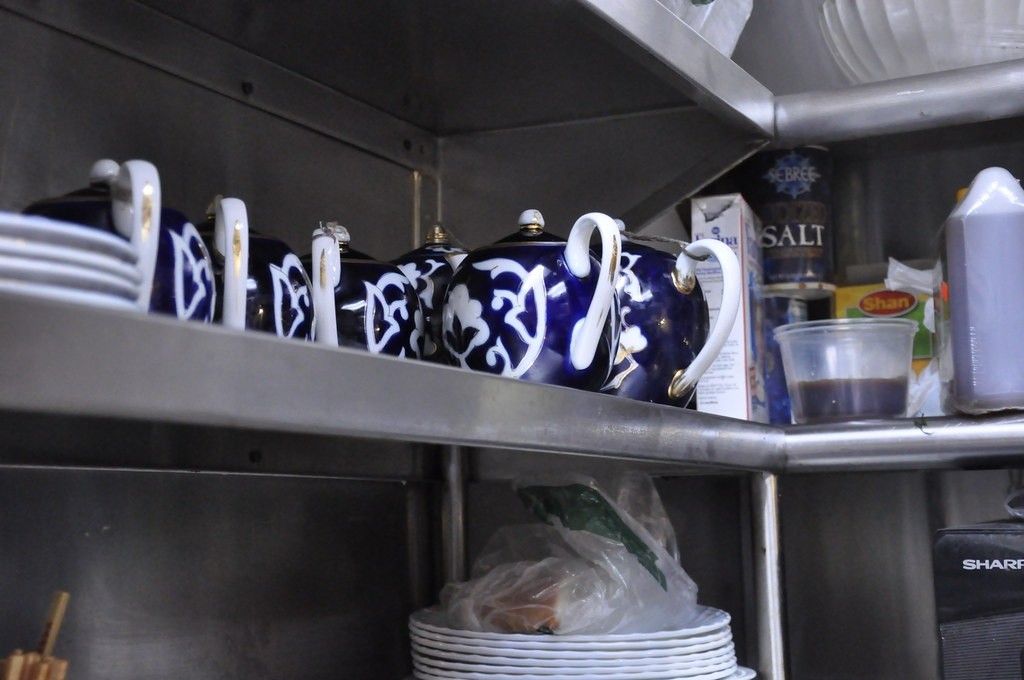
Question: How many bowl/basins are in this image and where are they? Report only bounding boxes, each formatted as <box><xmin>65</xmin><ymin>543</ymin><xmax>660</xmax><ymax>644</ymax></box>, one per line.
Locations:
<box><xmin>773</xmin><ymin>318</ymin><xmax>919</xmax><ymax>423</ymax></box>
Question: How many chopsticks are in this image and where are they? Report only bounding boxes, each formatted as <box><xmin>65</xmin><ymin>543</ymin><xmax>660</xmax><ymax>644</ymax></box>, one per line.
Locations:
<box><xmin>0</xmin><ymin>591</ymin><xmax>71</xmax><ymax>680</ymax></box>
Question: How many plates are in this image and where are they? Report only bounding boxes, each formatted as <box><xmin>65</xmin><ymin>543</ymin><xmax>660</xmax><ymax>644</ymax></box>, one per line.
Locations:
<box><xmin>408</xmin><ymin>604</ymin><xmax>758</xmax><ymax>680</ymax></box>
<box><xmin>1</xmin><ymin>212</ymin><xmax>141</xmax><ymax>314</ymax></box>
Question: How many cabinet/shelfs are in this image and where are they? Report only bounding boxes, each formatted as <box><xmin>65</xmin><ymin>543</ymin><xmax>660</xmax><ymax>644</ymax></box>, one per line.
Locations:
<box><xmin>774</xmin><ymin>59</ymin><xmax>1024</xmax><ymax>680</ymax></box>
<box><xmin>0</xmin><ymin>0</ymin><xmax>788</xmax><ymax>680</ymax></box>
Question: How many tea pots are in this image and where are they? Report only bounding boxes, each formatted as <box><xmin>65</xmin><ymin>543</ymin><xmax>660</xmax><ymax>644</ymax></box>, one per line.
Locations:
<box><xmin>18</xmin><ymin>158</ymin><xmax>744</xmax><ymax>409</ymax></box>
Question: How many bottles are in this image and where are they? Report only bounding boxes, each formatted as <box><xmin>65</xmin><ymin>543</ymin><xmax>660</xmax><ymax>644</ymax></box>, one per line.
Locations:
<box><xmin>934</xmin><ymin>167</ymin><xmax>1024</xmax><ymax>414</ymax></box>
